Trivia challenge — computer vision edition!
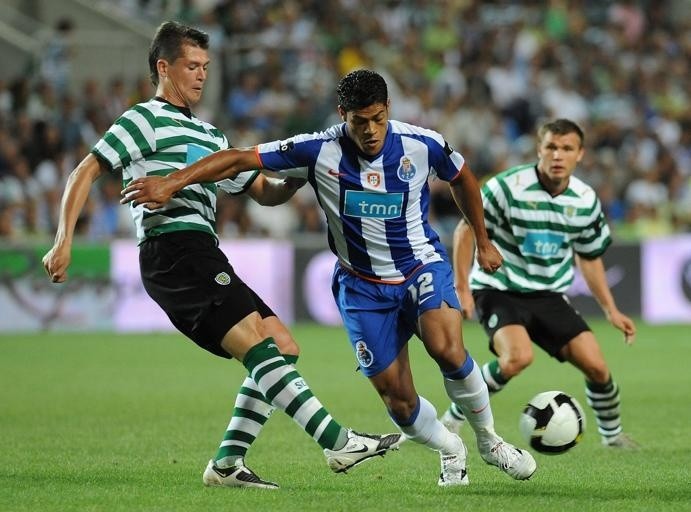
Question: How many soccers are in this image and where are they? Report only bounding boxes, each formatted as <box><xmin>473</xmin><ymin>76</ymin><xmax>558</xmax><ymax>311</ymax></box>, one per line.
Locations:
<box><xmin>517</xmin><ymin>391</ymin><xmax>584</xmax><ymax>455</ymax></box>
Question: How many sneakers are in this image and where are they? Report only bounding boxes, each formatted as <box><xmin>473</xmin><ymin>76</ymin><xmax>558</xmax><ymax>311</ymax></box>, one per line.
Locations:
<box><xmin>203</xmin><ymin>458</ymin><xmax>280</xmax><ymax>491</ymax></box>
<box><xmin>600</xmin><ymin>432</ymin><xmax>644</xmax><ymax>449</ymax></box>
<box><xmin>435</xmin><ymin>410</ymin><xmax>470</xmax><ymax>487</ymax></box>
<box><xmin>479</xmin><ymin>437</ymin><xmax>536</xmax><ymax>480</ymax></box>
<box><xmin>324</xmin><ymin>428</ymin><xmax>406</xmax><ymax>474</ymax></box>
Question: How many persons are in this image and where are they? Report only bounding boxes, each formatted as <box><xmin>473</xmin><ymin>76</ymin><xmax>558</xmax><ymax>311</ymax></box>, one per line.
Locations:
<box><xmin>445</xmin><ymin>113</ymin><xmax>640</xmax><ymax>451</ymax></box>
<box><xmin>116</xmin><ymin>68</ymin><xmax>539</xmax><ymax>488</ymax></box>
<box><xmin>37</xmin><ymin>20</ymin><xmax>410</xmax><ymax>488</ymax></box>
<box><xmin>2</xmin><ymin>0</ymin><xmax>691</xmax><ymax>242</ymax></box>
